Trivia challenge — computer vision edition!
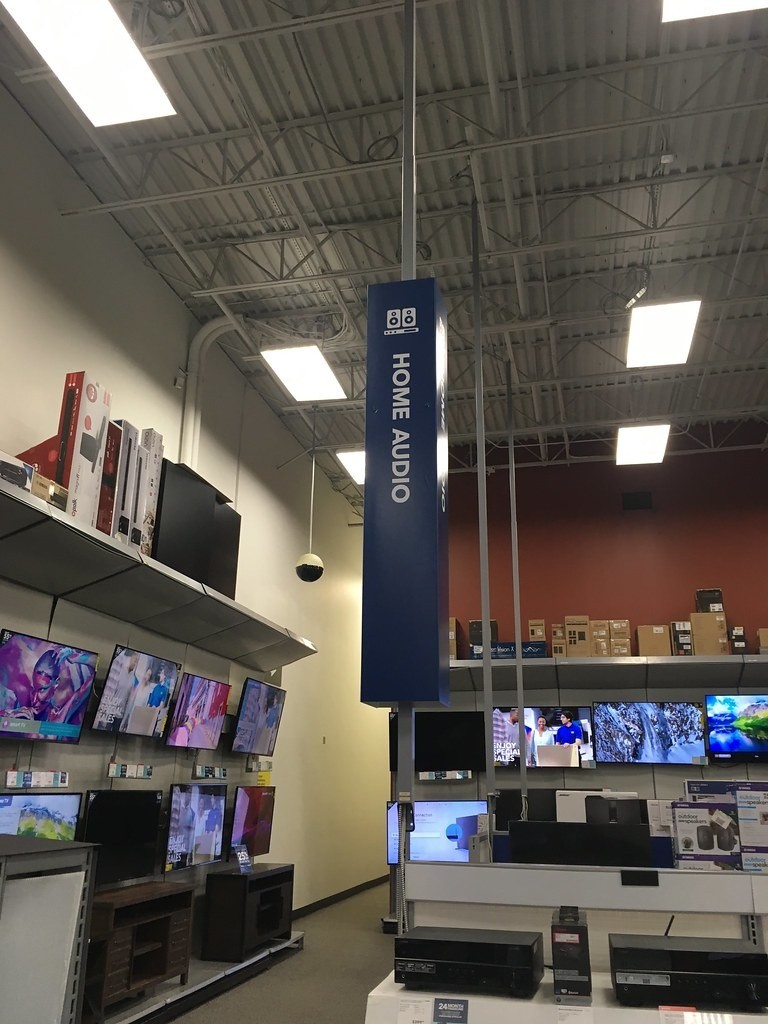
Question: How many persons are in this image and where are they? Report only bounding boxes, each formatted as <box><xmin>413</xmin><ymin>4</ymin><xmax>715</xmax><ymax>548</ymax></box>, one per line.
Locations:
<box><xmin>502</xmin><ymin>708</ymin><xmax>530</xmax><ymax>768</ymax></box>
<box><xmin>0</xmin><ymin>644</ymin><xmax>99</xmax><ymax>724</ymax></box>
<box><xmin>554</xmin><ymin>711</ymin><xmax>583</xmax><ymax>768</ymax></box>
<box><xmin>234</xmin><ymin>688</ymin><xmax>280</xmax><ymax>754</ymax></box>
<box><xmin>104</xmin><ymin>654</ymin><xmax>170</xmax><ymax>735</ymax></box>
<box><xmin>176</xmin><ymin>789</ymin><xmax>223</xmax><ymax>867</ymax></box>
<box><xmin>530</xmin><ymin>713</ymin><xmax>555</xmax><ymax>767</ymax></box>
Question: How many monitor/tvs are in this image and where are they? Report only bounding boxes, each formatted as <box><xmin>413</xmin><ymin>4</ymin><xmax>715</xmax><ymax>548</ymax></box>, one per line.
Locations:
<box><xmin>0</xmin><ymin>628</ymin><xmax>286</xmax><ymax>896</ymax></box>
<box><xmin>385</xmin><ymin>694</ymin><xmax>768</xmax><ymax>865</ymax></box>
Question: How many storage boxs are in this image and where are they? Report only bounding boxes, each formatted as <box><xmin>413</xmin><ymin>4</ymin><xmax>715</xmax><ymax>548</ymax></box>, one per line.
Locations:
<box><xmin>0</xmin><ymin>367</ymin><xmax>166</xmax><ymax>556</ymax></box>
<box><xmin>670</xmin><ymin>778</ymin><xmax>768</xmax><ymax>874</ymax></box>
<box><xmin>445</xmin><ymin>590</ymin><xmax>768</xmax><ymax>659</ymax></box>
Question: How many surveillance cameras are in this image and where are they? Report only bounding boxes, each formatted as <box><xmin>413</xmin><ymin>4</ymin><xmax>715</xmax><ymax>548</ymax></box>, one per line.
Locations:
<box><xmin>296</xmin><ymin>554</ymin><xmax>324</xmax><ymax>583</ymax></box>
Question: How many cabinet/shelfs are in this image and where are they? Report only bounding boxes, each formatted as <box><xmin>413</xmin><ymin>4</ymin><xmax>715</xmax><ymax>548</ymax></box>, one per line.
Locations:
<box><xmin>0</xmin><ymin>479</ymin><xmax>316</xmax><ymax>1024</ymax></box>
<box><xmin>365</xmin><ymin>650</ymin><xmax>768</xmax><ymax>1024</ymax></box>
